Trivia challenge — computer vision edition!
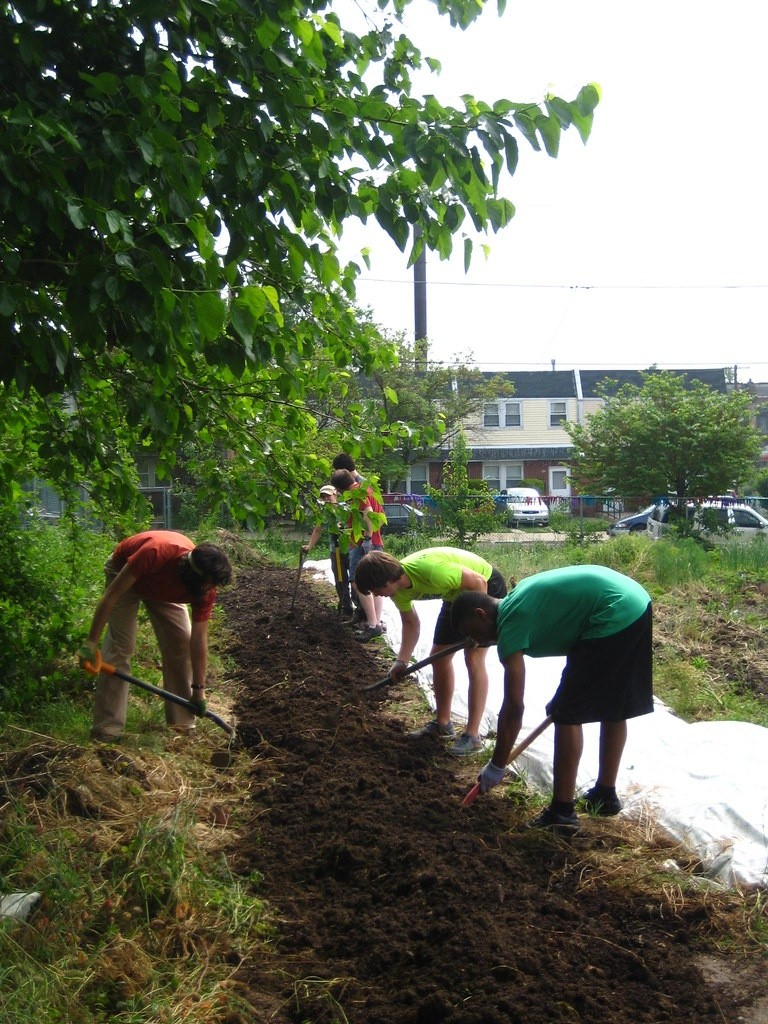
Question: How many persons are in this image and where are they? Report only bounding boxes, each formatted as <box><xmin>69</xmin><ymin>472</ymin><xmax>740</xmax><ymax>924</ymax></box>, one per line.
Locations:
<box><xmin>78</xmin><ymin>529</ymin><xmax>232</xmax><ymax>736</ymax></box>
<box><xmin>451</xmin><ymin>565</ymin><xmax>654</xmax><ymax>836</ymax></box>
<box><xmin>300</xmin><ymin>453</ymin><xmax>388</xmax><ymax>643</ymax></box>
<box><xmin>355</xmin><ymin>546</ymin><xmax>507</xmax><ymax>756</ymax></box>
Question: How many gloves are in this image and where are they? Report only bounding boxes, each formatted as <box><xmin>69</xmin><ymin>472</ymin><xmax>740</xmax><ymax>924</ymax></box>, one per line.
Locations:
<box><xmin>79</xmin><ymin>640</ymin><xmax>96</xmax><ymax>669</ymax></box>
<box><xmin>388</xmin><ymin>659</ymin><xmax>407</xmax><ymax>686</ymax></box>
<box><xmin>478</xmin><ymin>760</ymin><xmax>505</xmax><ymax>794</ymax></box>
<box><xmin>362</xmin><ymin>538</ymin><xmax>373</xmax><ymax>554</ymax></box>
<box><xmin>190</xmin><ymin>695</ymin><xmax>208</xmax><ymax>719</ymax></box>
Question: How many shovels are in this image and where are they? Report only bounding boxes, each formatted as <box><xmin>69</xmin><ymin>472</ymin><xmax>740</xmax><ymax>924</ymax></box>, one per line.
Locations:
<box><xmin>332</xmin><ymin>535</ymin><xmax>343</xmax><ymax>616</ymax></box>
<box><xmin>84</xmin><ymin>648</ymin><xmax>237</xmax><ymax>744</ymax></box>
<box><xmin>287</xmin><ymin>547</ymin><xmax>308</xmax><ymax>615</ymax></box>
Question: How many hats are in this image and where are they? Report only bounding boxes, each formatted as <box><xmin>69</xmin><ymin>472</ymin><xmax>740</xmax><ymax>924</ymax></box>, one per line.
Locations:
<box><xmin>320</xmin><ymin>485</ymin><xmax>336</xmax><ymax>495</ymax></box>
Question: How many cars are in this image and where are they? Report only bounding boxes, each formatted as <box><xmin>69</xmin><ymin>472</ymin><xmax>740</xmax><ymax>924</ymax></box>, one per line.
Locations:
<box><xmin>497</xmin><ymin>488</ymin><xmax>549</xmax><ymax>527</ymax></box>
<box><xmin>380</xmin><ymin>503</ymin><xmax>424</xmax><ymax>533</ymax></box>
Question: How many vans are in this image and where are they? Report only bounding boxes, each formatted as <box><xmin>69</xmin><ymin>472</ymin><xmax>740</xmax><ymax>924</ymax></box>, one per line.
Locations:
<box><xmin>606</xmin><ymin>495</ymin><xmax>735</xmax><ymax>539</ymax></box>
<box><xmin>646</xmin><ymin>501</ymin><xmax>768</xmax><ymax>546</ymax></box>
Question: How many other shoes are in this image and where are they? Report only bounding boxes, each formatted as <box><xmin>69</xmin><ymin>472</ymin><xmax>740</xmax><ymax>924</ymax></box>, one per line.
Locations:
<box><xmin>519</xmin><ymin>808</ymin><xmax>579</xmax><ymax>835</ymax></box>
<box><xmin>573</xmin><ymin>782</ymin><xmax>622</xmax><ymax>816</ymax></box>
<box><xmin>407</xmin><ymin>720</ymin><xmax>457</xmax><ymax>738</ymax></box>
<box><xmin>449</xmin><ymin>733</ymin><xmax>484</xmax><ymax>757</ymax></box>
<box><xmin>339</xmin><ymin>612</ymin><xmax>387</xmax><ymax>642</ymax></box>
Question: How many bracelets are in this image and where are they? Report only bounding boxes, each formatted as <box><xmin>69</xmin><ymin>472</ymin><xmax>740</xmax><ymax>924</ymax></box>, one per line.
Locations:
<box><xmin>191</xmin><ymin>683</ymin><xmax>205</xmax><ymax>689</ymax></box>
